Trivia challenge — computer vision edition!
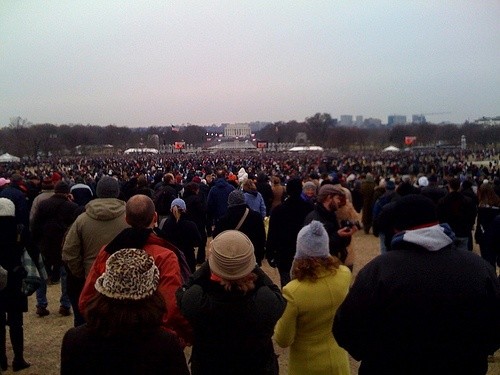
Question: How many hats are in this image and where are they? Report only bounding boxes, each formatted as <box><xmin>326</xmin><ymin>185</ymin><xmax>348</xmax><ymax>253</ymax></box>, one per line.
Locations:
<box><xmin>228</xmin><ymin>190</ymin><xmax>245</xmax><ymax>203</ymax></box>
<box><xmin>55</xmin><ymin>181</ymin><xmax>70</xmax><ymax>195</ymax></box>
<box><xmin>393</xmin><ymin>194</ymin><xmax>439</xmax><ymax>232</ymax></box>
<box><xmin>0</xmin><ymin>197</ymin><xmax>16</xmax><ymax>217</ymax></box>
<box><xmin>209</xmin><ymin>229</ymin><xmax>257</xmax><ymax>279</ymax></box>
<box><xmin>287</xmin><ymin>178</ymin><xmax>303</xmax><ymax>197</ymax></box>
<box><xmin>290</xmin><ymin>219</ymin><xmax>331</xmax><ymax>280</ymax></box>
<box><xmin>169</xmin><ymin>197</ymin><xmax>186</xmax><ymax>212</ymax></box>
<box><xmin>93</xmin><ymin>248</ymin><xmax>160</xmax><ymax>298</ymax></box>
<box><xmin>318</xmin><ymin>184</ymin><xmax>346</xmax><ymax>195</ymax></box>
<box><xmin>0</xmin><ymin>177</ymin><xmax>10</xmax><ymax>186</ymax></box>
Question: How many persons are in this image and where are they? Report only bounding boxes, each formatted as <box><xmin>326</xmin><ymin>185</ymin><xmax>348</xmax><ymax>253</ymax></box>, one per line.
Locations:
<box><xmin>0</xmin><ymin>148</ymin><xmax>500</xmax><ymax>375</ymax></box>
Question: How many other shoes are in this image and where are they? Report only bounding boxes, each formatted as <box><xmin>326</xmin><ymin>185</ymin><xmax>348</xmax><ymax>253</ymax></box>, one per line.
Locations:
<box><xmin>58</xmin><ymin>305</ymin><xmax>72</xmax><ymax>315</ymax></box>
<box><xmin>36</xmin><ymin>305</ymin><xmax>50</xmax><ymax>316</ymax></box>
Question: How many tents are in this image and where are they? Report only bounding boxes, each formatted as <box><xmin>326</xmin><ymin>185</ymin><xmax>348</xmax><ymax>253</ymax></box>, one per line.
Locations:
<box><xmin>385</xmin><ymin>146</ymin><xmax>400</xmax><ymax>151</ymax></box>
<box><xmin>0</xmin><ymin>153</ymin><xmax>20</xmax><ymax>163</ymax></box>
<box><xmin>290</xmin><ymin>145</ymin><xmax>324</xmax><ymax>152</ymax></box>
<box><xmin>124</xmin><ymin>148</ymin><xmax>159</xmax><ymax>154</ymax></box>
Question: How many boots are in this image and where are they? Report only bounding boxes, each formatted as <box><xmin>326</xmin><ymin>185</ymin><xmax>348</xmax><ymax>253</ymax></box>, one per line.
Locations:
<box><xmin>0</xmin><ymin>329</ymin><xmax>8</xmax><ymax>371</ymax></box>
<box><xmin>9</xmin><ymin>326</ymin><xmax>31</xmax><ymax>372</ymax></box>
